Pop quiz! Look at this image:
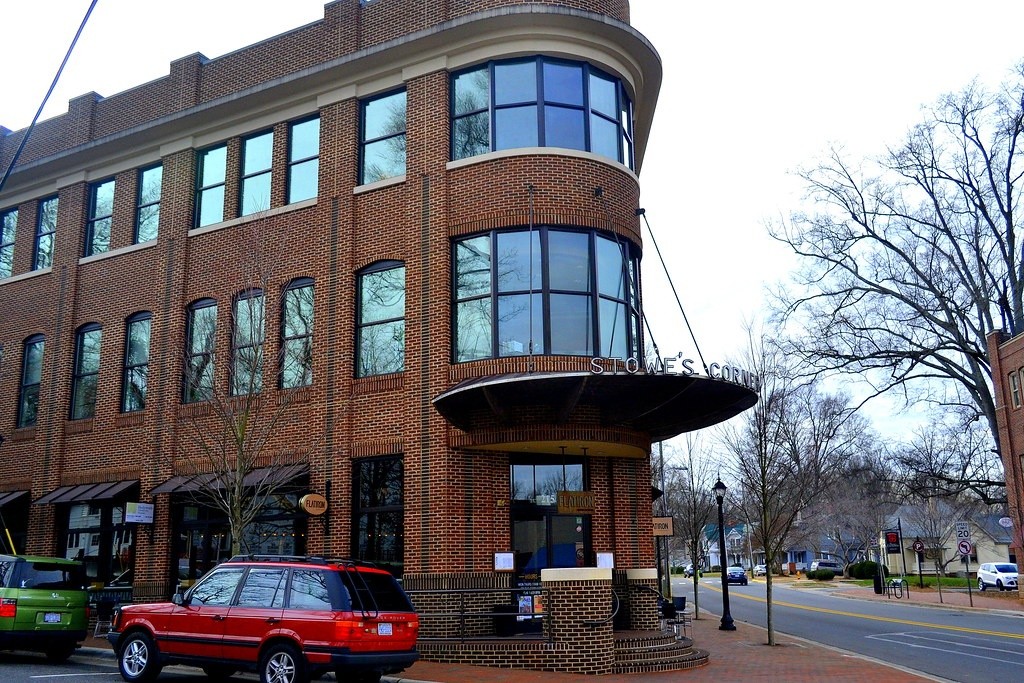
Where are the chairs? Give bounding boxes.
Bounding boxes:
[662,603,687,637]
[672,597,686,637]
[93,602,116,639]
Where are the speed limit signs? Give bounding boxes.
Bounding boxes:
[955,521,970,538]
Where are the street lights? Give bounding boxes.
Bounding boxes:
[663,466,689,599]
[712,469,736,631]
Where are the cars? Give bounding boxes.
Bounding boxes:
[726,568,748,585]
[684,564,702,578]
[751,565,767,575]
[178,559,203,578]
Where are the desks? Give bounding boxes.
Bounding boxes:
[658,611,694,638]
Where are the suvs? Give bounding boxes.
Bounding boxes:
[977,562,1019,591]
[94,554,421,683]
[0,554,90,661]
[810,558,842,574]
